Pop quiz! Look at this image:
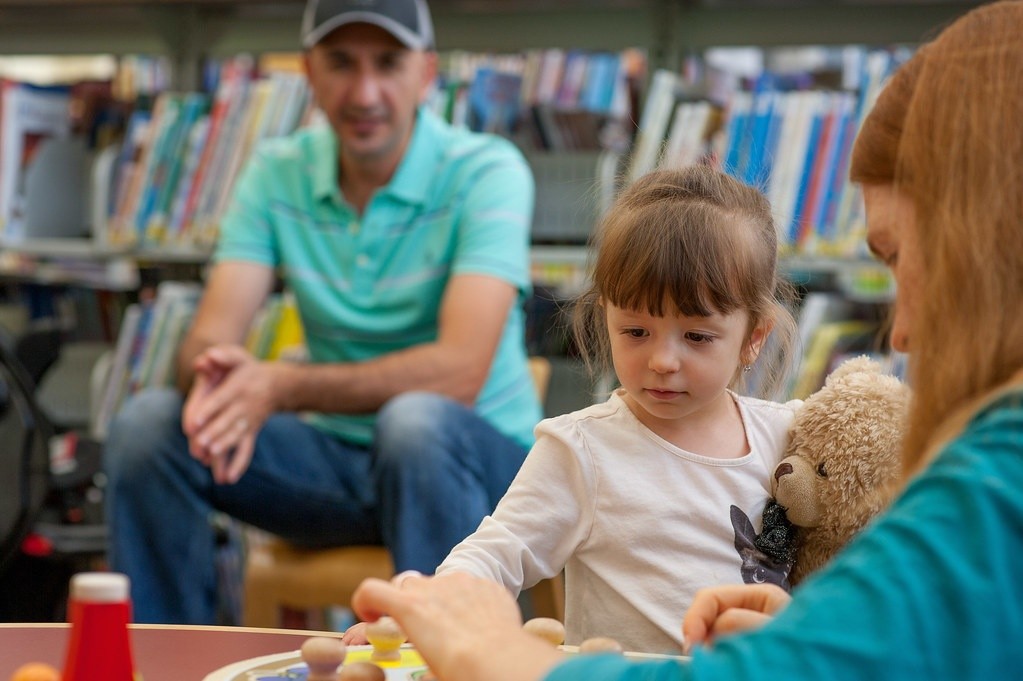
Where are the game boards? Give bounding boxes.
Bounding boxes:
[202,637,694,681]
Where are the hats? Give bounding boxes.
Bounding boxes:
[300,1,438,56]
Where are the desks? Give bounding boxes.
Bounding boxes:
[0,622,693,681]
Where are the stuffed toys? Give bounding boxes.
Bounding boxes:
[758,354,911,583]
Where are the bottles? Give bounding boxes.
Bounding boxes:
[60,572,135,681]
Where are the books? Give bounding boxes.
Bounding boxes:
[427,41,906,403]
[0,55,317,437]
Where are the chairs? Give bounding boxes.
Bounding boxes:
[241,357,564,629]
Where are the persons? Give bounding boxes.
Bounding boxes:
[104,0,544,624]
[353,0,1023,681]
[342,166,807,656]
